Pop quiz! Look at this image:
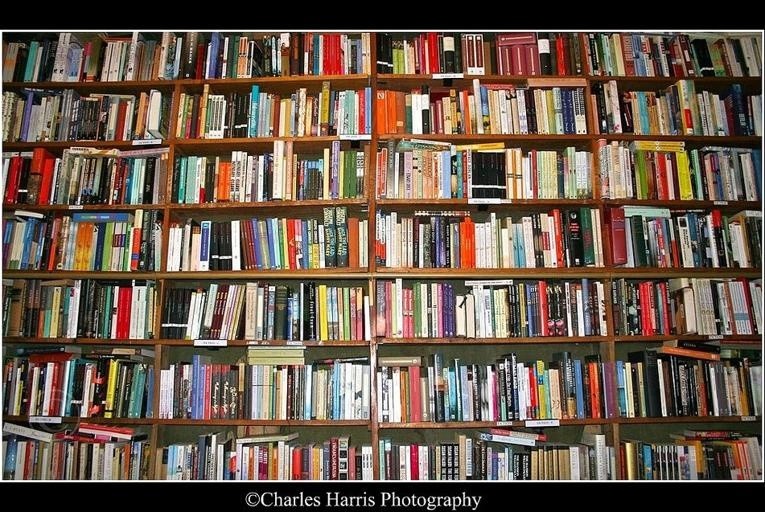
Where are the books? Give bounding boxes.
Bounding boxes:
[584,34,762,76]
[2,279,157,341]
[2,209,162,272]
[183,32,371,79]
[160,281,371,343]
[375,207,604,268]
[154,427,373,479]
[616,341,761,418]
[378,77,588,134]
[590,78,762,138]
[600,139,761,200]
[374,279,607,337]
[166,207,368,271]
[376,138,593,201]
[173,141,370,205]
[2,33,183,82]
[2,147,169,205]
[604,205,762,268]
[2,88,170,140]
[375,352,609,422]
[612,276,762,338]
[175,80,371,138]
[2,421,150,480]
[379,428,616,482]
[2,344,153,418]
[620,429,763,480]
[377,33,586,73]
[159,345,371,421]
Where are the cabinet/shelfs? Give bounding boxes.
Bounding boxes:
[2,28,761,480]
